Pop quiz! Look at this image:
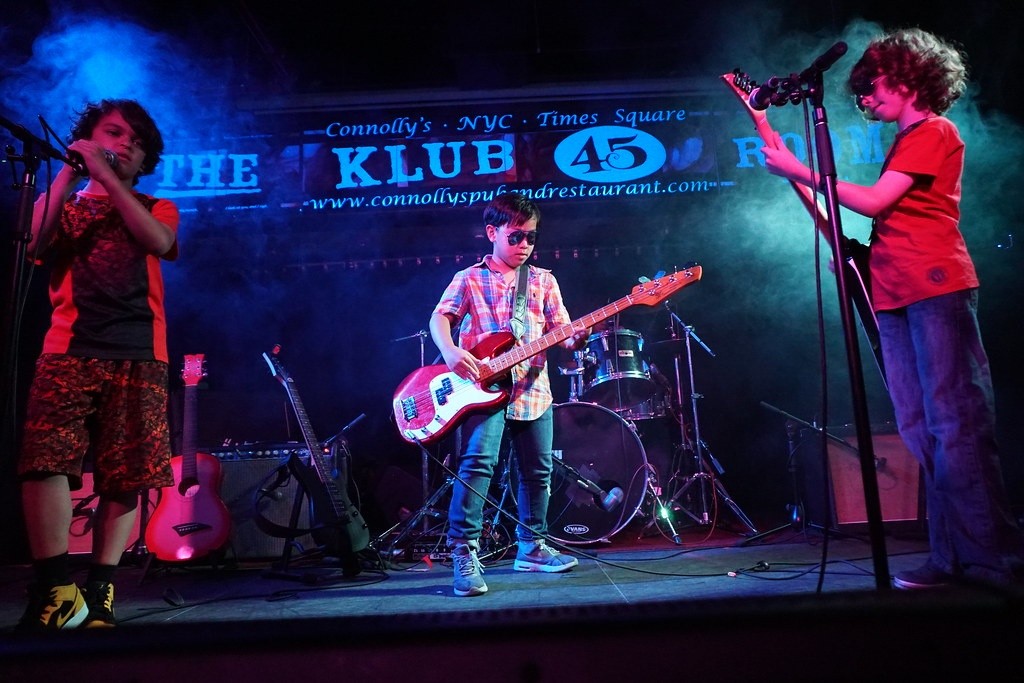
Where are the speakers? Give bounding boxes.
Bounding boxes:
[204,442,337,563]
[63,472,142,557]
[799,421,929,535]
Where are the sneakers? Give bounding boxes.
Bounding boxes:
[16,582,89,631]
[78,580,116,630]
[451,544,488,596]
[514,539,578,572]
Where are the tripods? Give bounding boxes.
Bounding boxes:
[636,296,874,546]
[381,343,600,564]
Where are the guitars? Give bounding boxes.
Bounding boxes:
[391,261,703,445]
[145,352,232,562]
[262,348,371,556]
[720,67,888,393]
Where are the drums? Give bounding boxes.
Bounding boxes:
[576,328,657,411]
[506,402,650,544]
[620,376,684,422]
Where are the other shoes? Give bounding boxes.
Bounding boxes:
[893,557,947,588]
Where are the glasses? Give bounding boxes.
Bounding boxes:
[496,227,537,246]
[857,74,887,96]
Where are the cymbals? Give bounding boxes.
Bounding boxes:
[645,338,703,360]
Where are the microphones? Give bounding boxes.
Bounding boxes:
[71,507,97,517]
[649,363,671,388]
[596,488,626,513]
[65,147,119,169]
[749,77,779,111]
[607,298,616,330]
[258,488,282,501]
[875,459,889,472]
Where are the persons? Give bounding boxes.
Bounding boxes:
[15,98,178,639]
[760,28,1024,598]
[428,193,592,597]
